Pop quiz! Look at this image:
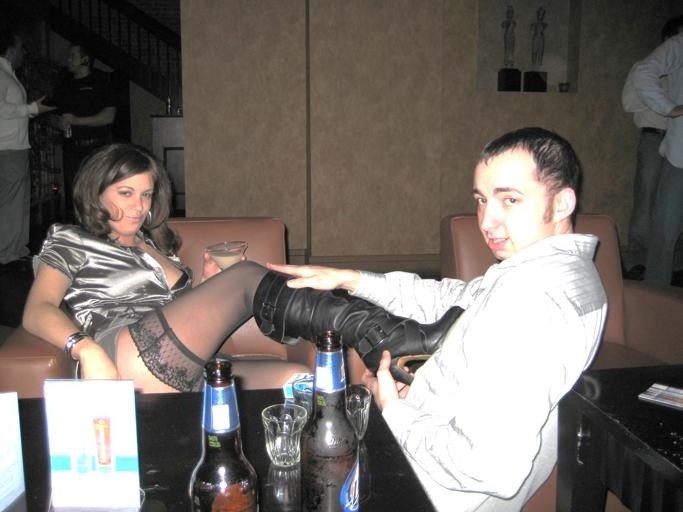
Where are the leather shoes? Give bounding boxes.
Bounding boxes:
[622,264,646,280]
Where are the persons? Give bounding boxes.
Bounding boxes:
[528,7,549,66]
[622,17,683,280]
[266,127,609,512]
[500,4,517,67]
[0,33,57,271]
[22,140,463,394]
[633,33,683,286]
[50,33,117,202]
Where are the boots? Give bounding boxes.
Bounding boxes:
[253,270,464,385]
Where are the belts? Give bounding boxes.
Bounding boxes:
[643,127,666,136]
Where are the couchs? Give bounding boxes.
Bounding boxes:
[439,209,683,511]
[0,215,372,402]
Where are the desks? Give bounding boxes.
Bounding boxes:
[0,379,441,511]
[553,362,683,511]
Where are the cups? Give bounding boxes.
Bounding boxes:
[346,382,371,440]
[265,462,300,511]
[359,441,371,504]
[206,240,248,270]
[291,377,313,418]
[559,83,570,92]
[261,404,306,466]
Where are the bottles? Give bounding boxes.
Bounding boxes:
[297,330,360,511]
[187,360,260,511]
[176,104,182,116]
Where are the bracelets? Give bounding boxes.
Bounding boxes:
[67,333,88,359]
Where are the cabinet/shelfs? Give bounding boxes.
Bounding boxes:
[148,114,185,217]
